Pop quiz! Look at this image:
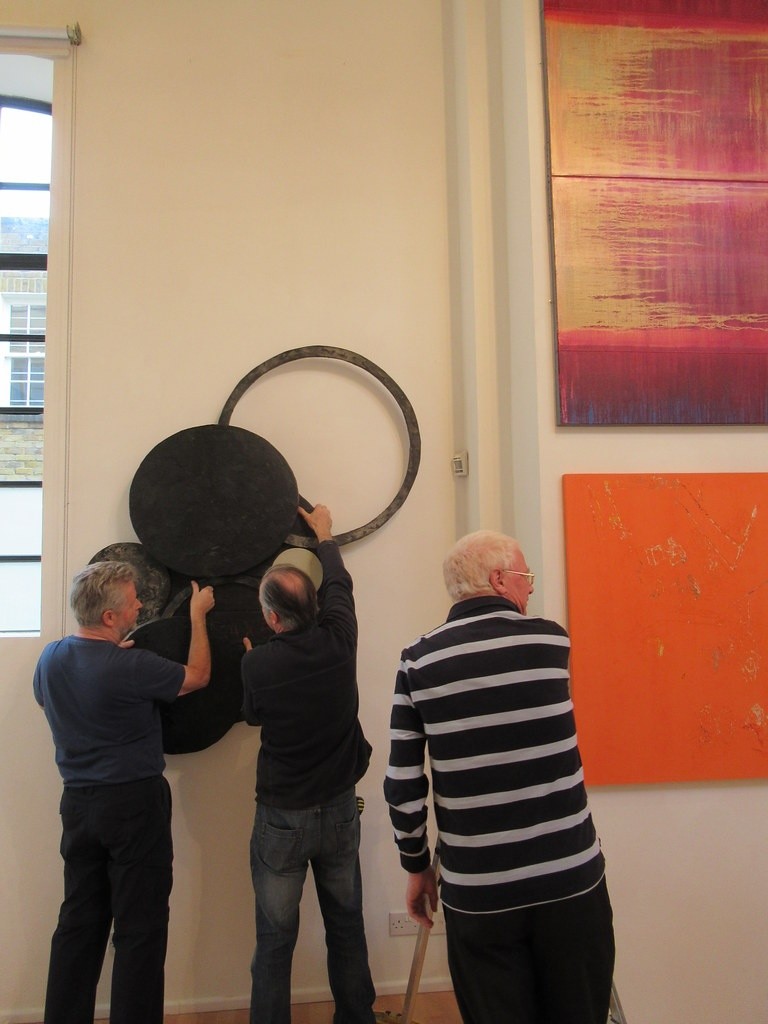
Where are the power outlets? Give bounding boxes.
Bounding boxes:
[388,911,419,936]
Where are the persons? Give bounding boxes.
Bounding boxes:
[383,531,616,1024]
[239,503,377,1024]
[34,561,216,1024]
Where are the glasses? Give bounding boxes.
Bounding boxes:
[504,569,535,585]
